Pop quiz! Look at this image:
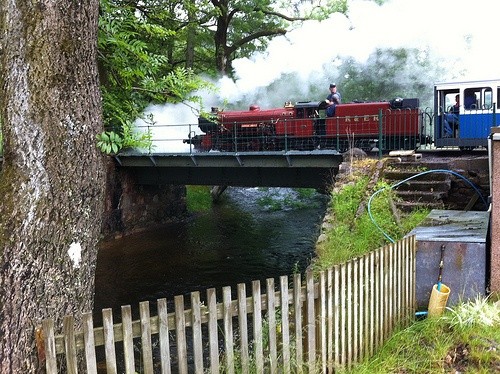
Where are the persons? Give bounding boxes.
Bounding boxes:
[325,82,341,118]
[450,95,460,114]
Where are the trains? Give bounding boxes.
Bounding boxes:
[181,79,500,154]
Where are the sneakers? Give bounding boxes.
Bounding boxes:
[443,134,447,138]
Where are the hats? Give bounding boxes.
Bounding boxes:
[329,82,336,88]
